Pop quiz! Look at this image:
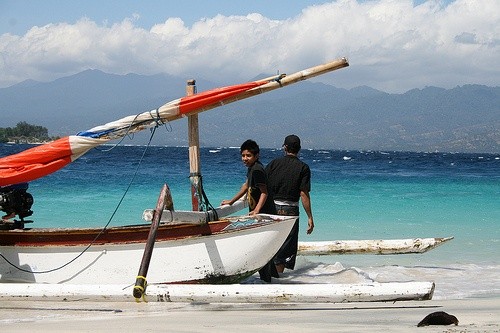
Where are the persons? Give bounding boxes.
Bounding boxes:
[220,140,279,284]
[264,135,314,273]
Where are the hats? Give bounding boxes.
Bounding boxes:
[281,135,301,148]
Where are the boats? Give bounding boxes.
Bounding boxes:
[0,56,350,286]
